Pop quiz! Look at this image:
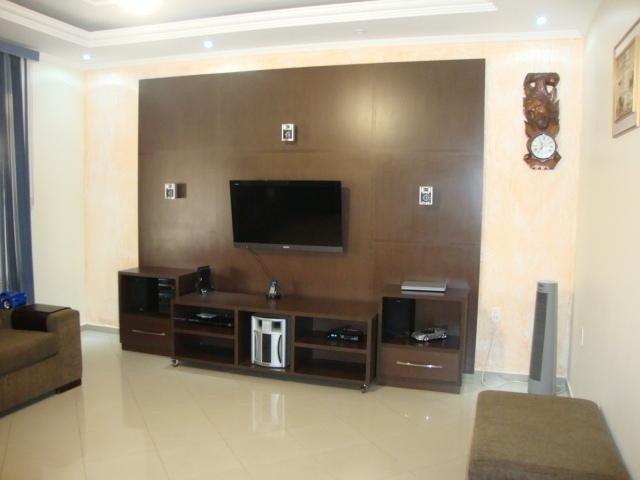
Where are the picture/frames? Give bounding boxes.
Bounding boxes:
[607,14,640,140]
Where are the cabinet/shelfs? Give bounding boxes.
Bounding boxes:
[373,278,470,395]
[169,289,380,394]
[116,265,201,356]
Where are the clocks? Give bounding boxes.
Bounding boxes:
[522,69,561,173]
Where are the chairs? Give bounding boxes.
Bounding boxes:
[1,301,83,420]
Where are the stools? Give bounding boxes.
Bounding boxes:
[467,387,632,479]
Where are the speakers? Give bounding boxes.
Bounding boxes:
[250,314,290,368]
[527,279,558,395]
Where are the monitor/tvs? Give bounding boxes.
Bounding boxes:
[229,180,344,253]
